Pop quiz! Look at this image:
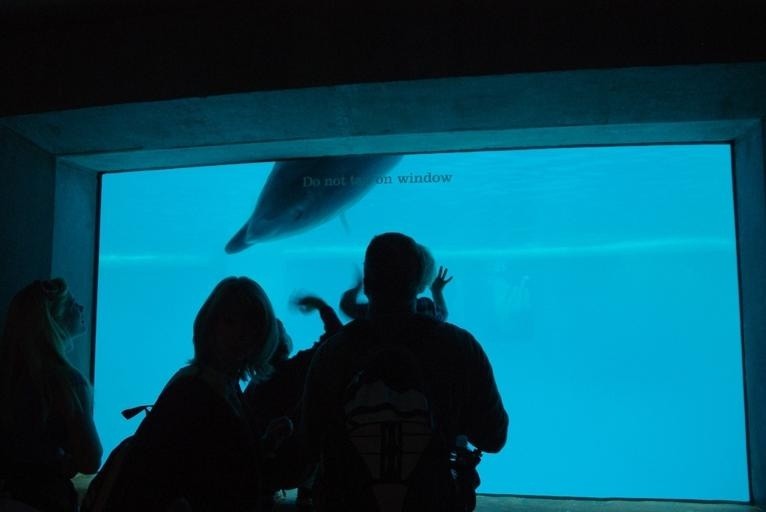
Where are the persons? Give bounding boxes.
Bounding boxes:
[301,233,508,512]
[243,297,343,511]
[1,277,103,511]
[340,268,451,322]
[107,278,280,511]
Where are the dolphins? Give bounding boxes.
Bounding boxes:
[225,151,406,253]
